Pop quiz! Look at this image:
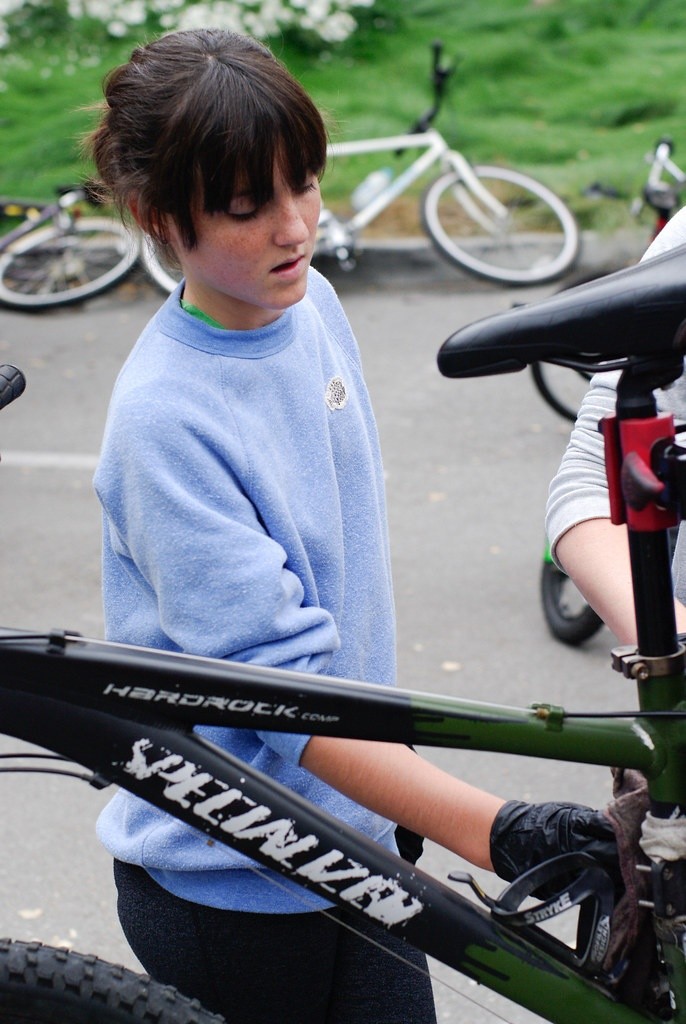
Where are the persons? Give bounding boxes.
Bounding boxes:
[545,207,686,647]
[90,29,620,1024]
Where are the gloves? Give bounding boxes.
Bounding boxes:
[489,797,624,904]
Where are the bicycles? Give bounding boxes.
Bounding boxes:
[0,182,143,310]
[530,137,685,425]
[1,242,685,1022]
[140,42,583,299]
